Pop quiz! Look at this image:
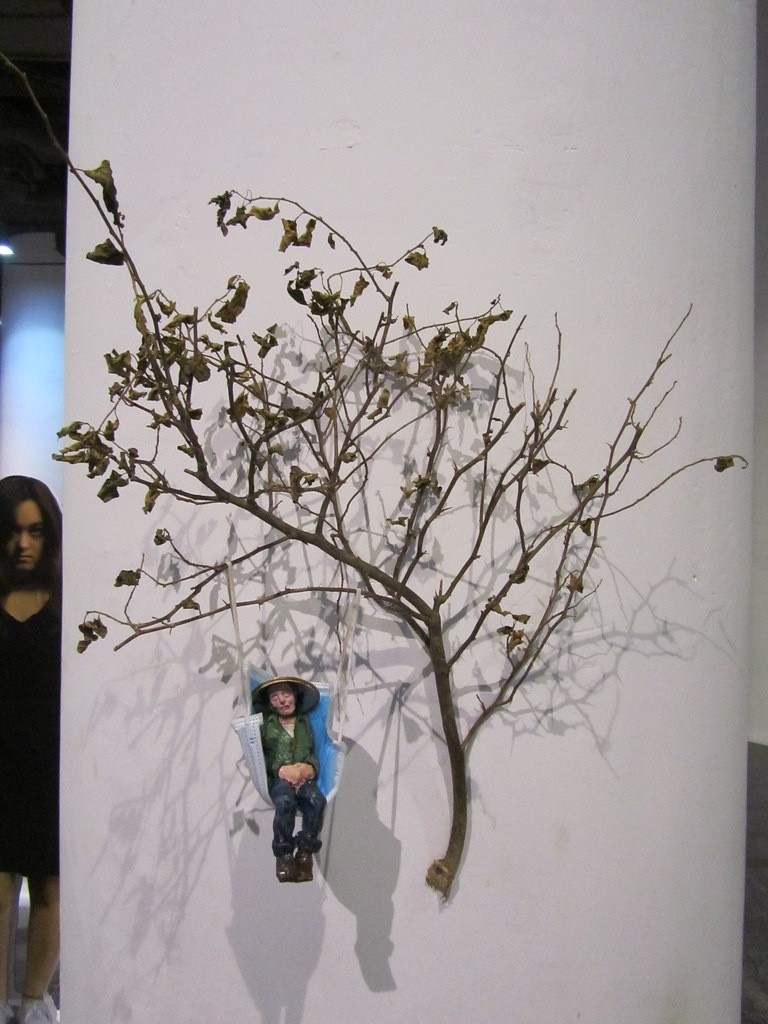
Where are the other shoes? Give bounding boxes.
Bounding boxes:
[21,992,57,1024]
[0,1000,16,1024]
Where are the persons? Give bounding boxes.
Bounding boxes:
[0,476,62,1024]
[253,676,327,882]
